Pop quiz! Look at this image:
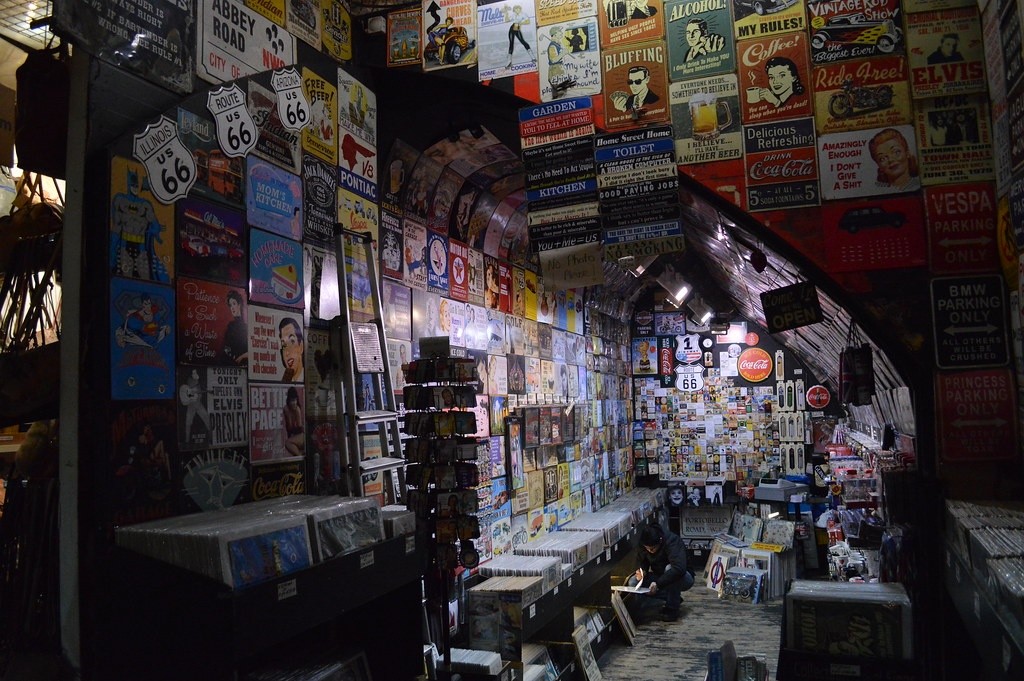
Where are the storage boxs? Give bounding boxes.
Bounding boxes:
[110,526,425,664]
[754,483,810,501]
[776,578,926,681]
[940,527,1024,681]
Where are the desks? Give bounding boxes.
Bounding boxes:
[747,495,831,572]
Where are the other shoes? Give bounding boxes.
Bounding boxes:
[662,613,677,622]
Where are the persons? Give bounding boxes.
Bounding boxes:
[637,523,696,621]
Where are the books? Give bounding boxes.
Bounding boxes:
[945,498,1024,630]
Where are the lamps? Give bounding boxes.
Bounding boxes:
[625,255,730,335]
